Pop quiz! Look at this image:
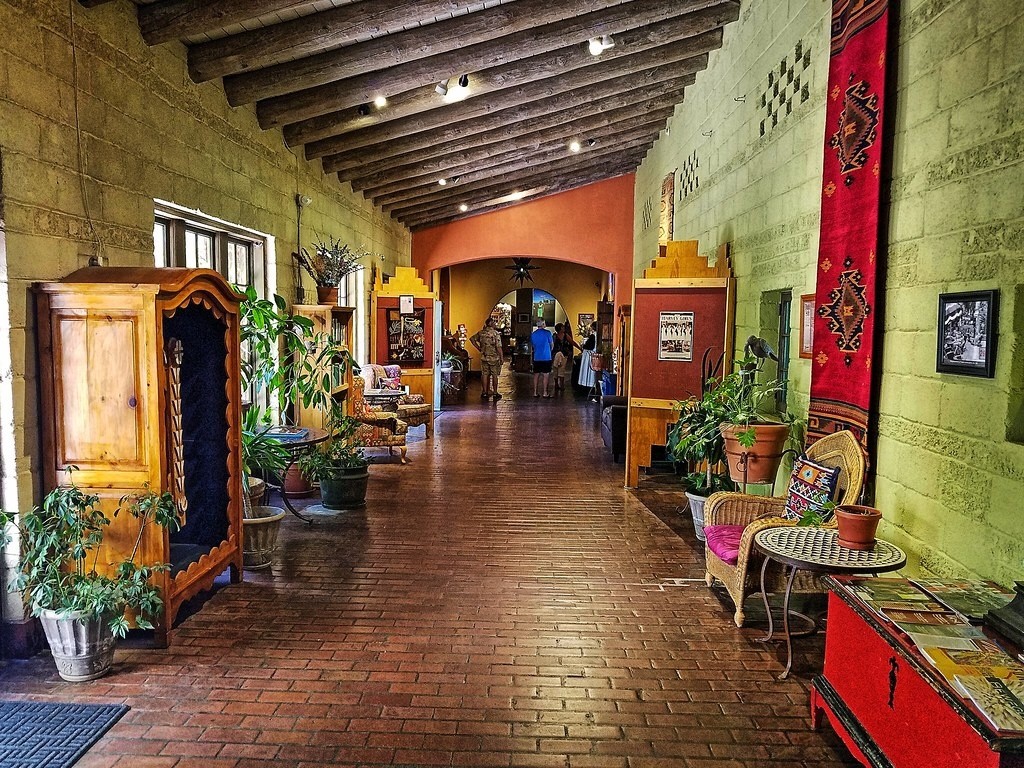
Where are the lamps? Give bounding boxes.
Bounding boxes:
[434,78,450,96]
[587,139,596,146]
[452,176,460,184]
[358,103,371,116]
[458,74,469,87]
[588,34,616,56]
[502,257,542,289]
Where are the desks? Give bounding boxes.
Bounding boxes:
[809,573,1024,768]
[255,425,330,526]
[362,388,407,414]
[753,525,907,680]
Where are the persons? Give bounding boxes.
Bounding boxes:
[530,317,554,397]
[553,323,582,393]
[470,318,503,398]
[662,323,692,337]
[577,322,597,388]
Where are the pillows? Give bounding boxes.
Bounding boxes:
[378,376,405,406]
[780,455,841,525]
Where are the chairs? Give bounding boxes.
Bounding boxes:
[702,430,866,629]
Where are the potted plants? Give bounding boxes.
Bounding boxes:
[0,464,182,684]
[230,233,370,572]
[665,345,727,541]
[591,346,612,369]
[799,492,883,551]
[666,334,812,492]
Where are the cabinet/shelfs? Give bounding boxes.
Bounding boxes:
[290,304,358,455]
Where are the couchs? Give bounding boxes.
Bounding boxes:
[353,363,432,464]
[600,395,629,465]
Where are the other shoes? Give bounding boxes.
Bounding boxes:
[493,393,502,399]
[550,391,558,397]
[481,393,489,400]
[533,392,539,397]
[543,393,549,397]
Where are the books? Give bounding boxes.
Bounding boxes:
[266,426,309,437]
[834,575,1024,734]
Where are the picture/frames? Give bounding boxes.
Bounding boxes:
[399,294,414,314]
[578,313,595,327]
[935,288,1003,379]
[657,310,694,362]
[518,312,531,324]
[798,293,817,359]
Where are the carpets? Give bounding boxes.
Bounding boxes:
[0,700,132,768]
